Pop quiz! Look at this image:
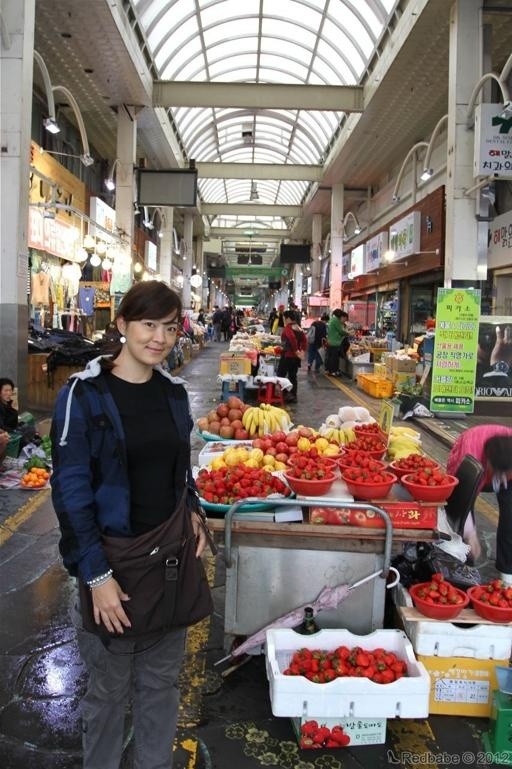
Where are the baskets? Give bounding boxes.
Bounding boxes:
[283,427,459,502]
[410,583,512,623]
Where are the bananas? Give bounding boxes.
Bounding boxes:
[241,403,292,438]
[327,429,356,446]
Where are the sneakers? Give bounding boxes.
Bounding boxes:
[328,369,341,376]
[501,570,512,583]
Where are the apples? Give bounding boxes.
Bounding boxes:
[198,396,248,440]
[253,427,313,461]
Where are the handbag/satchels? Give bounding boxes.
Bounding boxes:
[78,485,213,636]
[340,337,350,359]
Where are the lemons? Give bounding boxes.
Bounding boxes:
[211,446,286,472]
[22,467,50,487]
[296,436,342,455]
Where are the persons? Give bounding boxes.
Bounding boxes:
[477,323,512,388]
[50,280,214,769]
[446,424,512,588]
[0,378,18,432]
[198,306,348,404]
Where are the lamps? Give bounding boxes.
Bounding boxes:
[141,206,185,256]
[391,113,448,203]
[105,159,122,190]
[465,53,512,120]
[344,212,361,237]
[33,45,93,166]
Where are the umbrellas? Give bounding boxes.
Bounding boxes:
[213,566,400,668]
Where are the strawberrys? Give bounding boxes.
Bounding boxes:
[417,573,464,606]
[471,579,512,608]
[395,453,437,470]
[300,720,350,750]
[340,450,380,467]
[407,467,450,486]
[344,464,392,483]
[195,466,290,504]
[288,447,334,479]
[284,646,408,684]
[344,436,385,452]
[355,422,382,434]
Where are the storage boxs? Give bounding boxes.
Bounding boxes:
[290,717,387,750]
[264,628,429,720]
[393,580,512,661]
[357,353,416,398]
[219,357,251,374]
[310,502,437,530]
[419,657,509,718]
[39,418,53,439]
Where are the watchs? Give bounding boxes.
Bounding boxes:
[488,361,512,373]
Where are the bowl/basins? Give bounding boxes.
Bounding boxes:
[466,585,512,623]
[196,492,294,513]
[409,582,470,620]
[194,421,295,443]
[282,428,459,502]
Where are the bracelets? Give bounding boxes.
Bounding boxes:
[86,569,114,587]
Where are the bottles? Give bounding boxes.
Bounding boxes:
[302,606,319,635]
